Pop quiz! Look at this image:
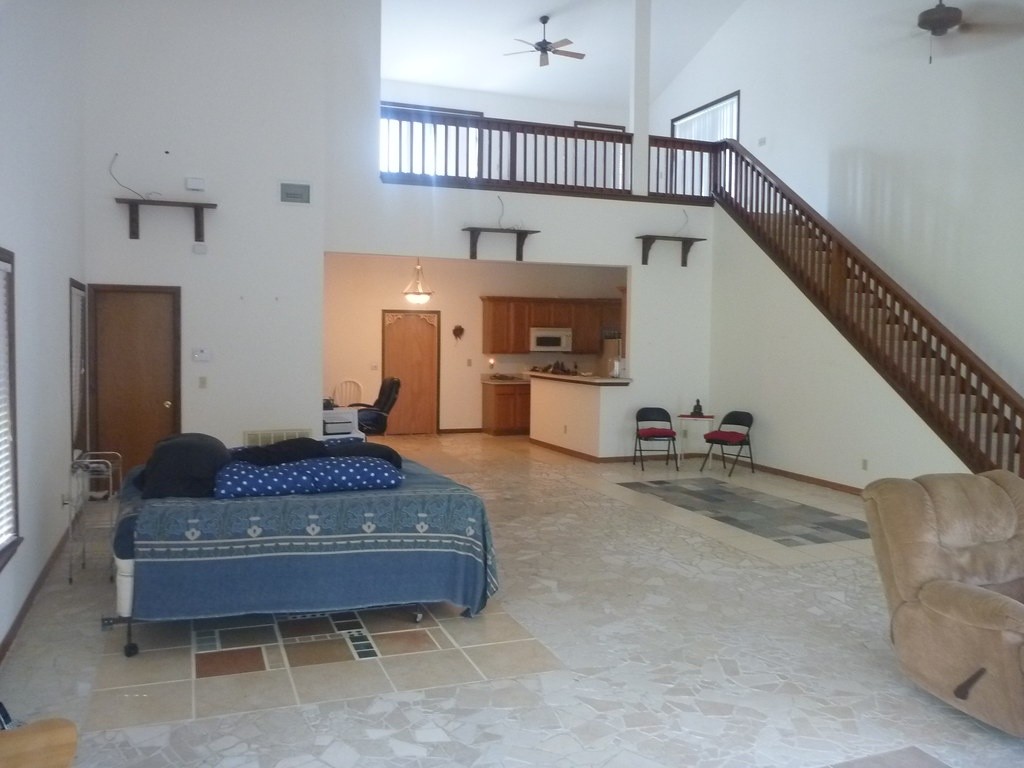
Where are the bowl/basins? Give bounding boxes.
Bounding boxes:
[603,328,621,338]
[522,372,531,381]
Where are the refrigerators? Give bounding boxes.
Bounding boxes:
[603,339,622,377]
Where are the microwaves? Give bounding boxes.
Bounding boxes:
[530,327,573,352]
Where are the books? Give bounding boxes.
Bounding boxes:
[325,416,352,434]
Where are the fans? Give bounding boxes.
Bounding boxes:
[505,16,585,67]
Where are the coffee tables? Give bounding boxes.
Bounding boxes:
[677,415,713,470]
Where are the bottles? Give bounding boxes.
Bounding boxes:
[574,359,578,374]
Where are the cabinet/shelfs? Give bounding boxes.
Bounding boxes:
[484,296,621,353]
[482,384,530,435]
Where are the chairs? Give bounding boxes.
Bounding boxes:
[632,407,681,470]
[335,377,400,435]
[860,471,1024,738]
[701,412,758,477]
[0,699,76,768]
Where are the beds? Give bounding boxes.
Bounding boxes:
[117,454,489,615]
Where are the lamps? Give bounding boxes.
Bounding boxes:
[402,256,434,305]
[916,0,961,36]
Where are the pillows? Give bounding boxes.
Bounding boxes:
[144,434,402,495]
